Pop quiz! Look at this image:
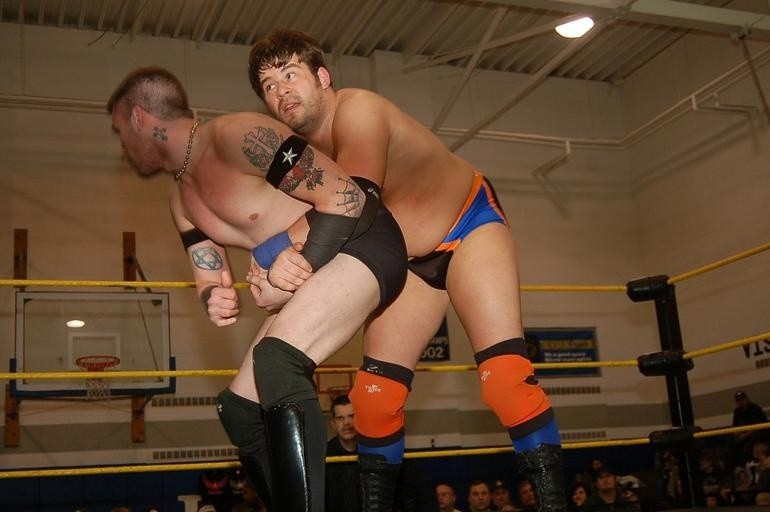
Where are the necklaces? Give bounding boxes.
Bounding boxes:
[173,121,200,182]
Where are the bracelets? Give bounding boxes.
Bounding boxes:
[251,229,295,273]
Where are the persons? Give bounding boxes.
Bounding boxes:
[730,389,769,448]
[247,30,578,512]
[324,393,364,512]
[437,479,536,510]
[201,470,266,510]
[105,65,408,511]
[573,444,768,511]
